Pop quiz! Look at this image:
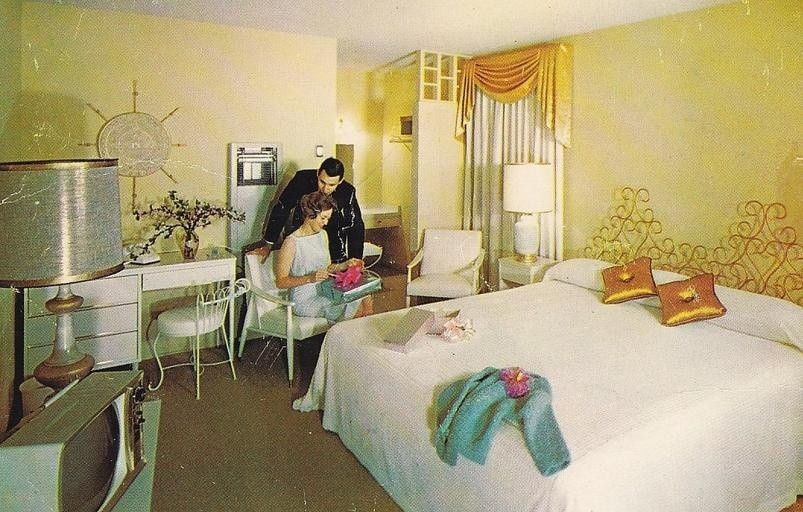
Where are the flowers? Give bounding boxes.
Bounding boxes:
[131,188,249,255]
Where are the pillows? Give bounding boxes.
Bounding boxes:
[600,255,726,327]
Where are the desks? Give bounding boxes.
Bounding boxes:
[0,397,164,512]
[2,244,237,386]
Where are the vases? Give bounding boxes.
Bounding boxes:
[177,229,198,260]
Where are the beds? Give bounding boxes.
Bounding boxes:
[326,254,803,512]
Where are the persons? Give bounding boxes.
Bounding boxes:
[276,190,373,325]
[244,157,365,264]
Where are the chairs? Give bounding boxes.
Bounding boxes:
[397,225,488,308]
[145,236,333,402]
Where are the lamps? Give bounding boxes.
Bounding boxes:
[498,160,555,263]
[1,156,126,413]
